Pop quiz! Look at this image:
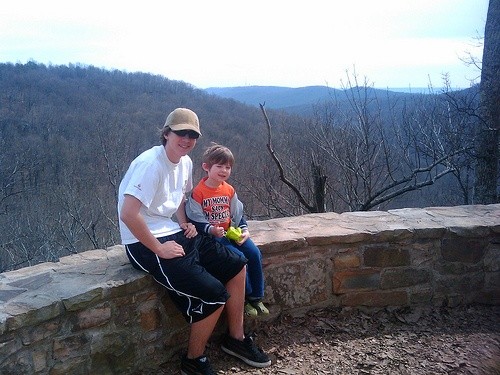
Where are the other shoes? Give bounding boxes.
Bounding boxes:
[244,303,257,317]
[181,350,218,375]
[221,330,271,367]
[256,301,270,317]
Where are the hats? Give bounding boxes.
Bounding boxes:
[163,108,203,137]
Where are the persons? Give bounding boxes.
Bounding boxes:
[118,108,271,375]
[186,145,269,317]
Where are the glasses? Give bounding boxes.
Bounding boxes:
[174,130,199,138]
